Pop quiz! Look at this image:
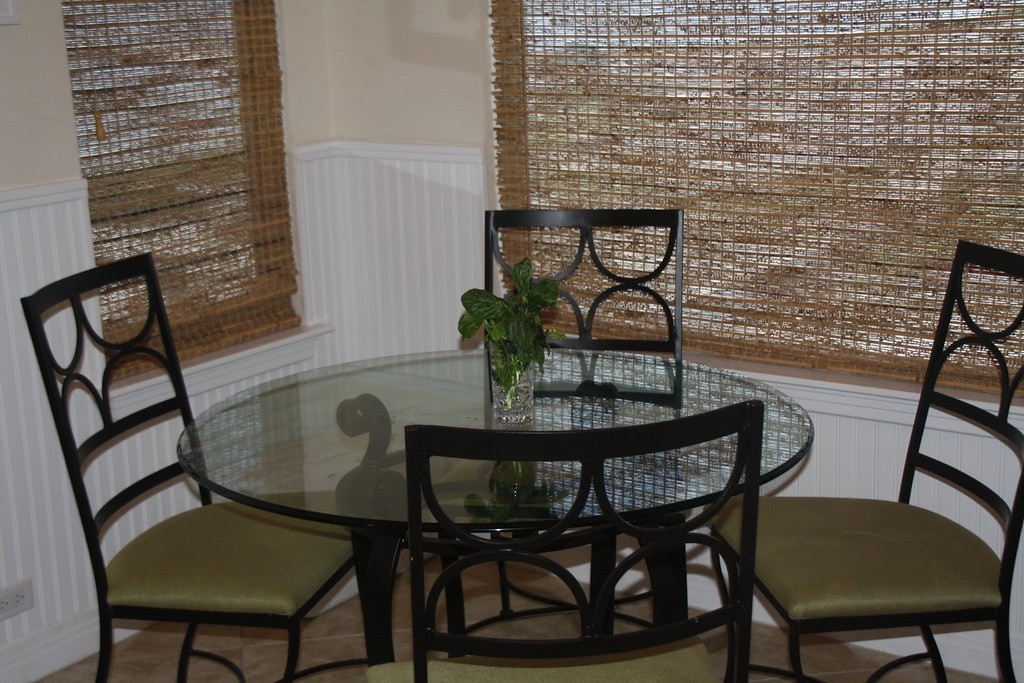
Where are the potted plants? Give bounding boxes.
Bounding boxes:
[455,259,564,421]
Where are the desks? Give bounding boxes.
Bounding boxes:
[181,344,813,683]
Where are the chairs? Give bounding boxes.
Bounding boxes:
[26,250,355,683]
[702,241,1023,683]
[358,396,763,683]
[466,204,684,357]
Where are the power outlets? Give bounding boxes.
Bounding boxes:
[1,578,35,623]
